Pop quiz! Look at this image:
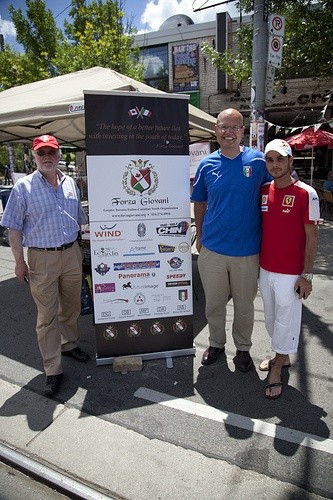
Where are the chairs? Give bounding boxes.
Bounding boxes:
[315,187,333,221]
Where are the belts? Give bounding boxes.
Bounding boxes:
[30,236,79,252]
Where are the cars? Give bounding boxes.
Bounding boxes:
[78,200,94,314]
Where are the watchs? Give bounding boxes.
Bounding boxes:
[300,272,313,281]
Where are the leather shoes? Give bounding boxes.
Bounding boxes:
[201,346,225,365]
[235,350,252,371]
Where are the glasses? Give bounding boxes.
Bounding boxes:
[216,123,244,132]
[36,150,56,156]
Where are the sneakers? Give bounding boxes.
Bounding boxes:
[43,373,64,395]
[61,347,89,362]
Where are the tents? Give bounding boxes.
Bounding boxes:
[1,64,218,154]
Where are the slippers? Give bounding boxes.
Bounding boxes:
[264,377,284,399]
[259,358,291,371]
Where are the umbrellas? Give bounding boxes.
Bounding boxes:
[283,127,332,150]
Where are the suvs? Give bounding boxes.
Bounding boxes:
[0,185,26,248]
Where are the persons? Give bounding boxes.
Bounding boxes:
[259,139,320,398]
[190,108,275,371]
[0,134,89,397]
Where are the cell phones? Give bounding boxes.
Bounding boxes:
[296,286,305,298]
[24,276,29,284]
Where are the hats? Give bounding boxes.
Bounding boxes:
[263,139,293,161]
[32,134,59,151]
